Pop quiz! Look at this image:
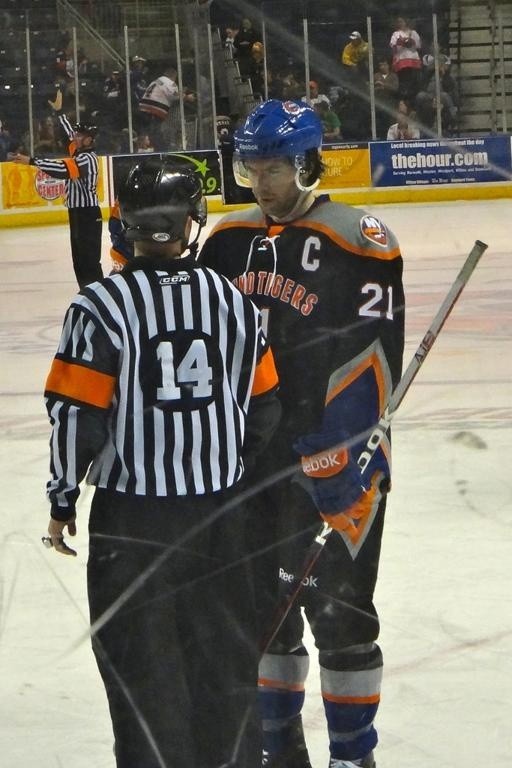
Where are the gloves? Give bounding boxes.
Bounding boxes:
[285,428,378,531]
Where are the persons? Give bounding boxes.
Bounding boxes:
[42,158,284,767]
[15,88,102,286]
[1,7,460,163]
[202,98,401,767]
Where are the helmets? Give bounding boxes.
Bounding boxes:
[232,97,324,164]
[72,121,98,135]
[117,152,210,244]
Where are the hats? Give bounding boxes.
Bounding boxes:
[348,31,361,41]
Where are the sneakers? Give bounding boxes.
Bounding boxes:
[259,747,313,768]
[328,749,378,768]
